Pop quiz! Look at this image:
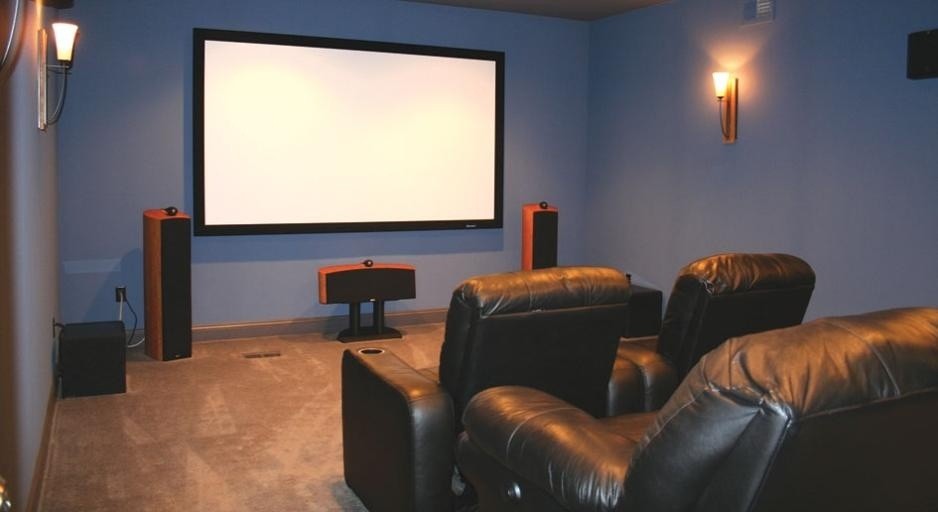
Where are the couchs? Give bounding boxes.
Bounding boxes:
[340,267,631,512]
[615,253,817,410]
[452,306,938,511]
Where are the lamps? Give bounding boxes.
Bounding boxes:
[713,69,738,144]
[37,20,79,130]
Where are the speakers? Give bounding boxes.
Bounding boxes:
[144,207,193,362]
[317,263,417,304]
[905,29,937,80]
[520,202,558,271]
[58,320,127,399]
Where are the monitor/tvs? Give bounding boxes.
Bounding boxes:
[191,23,507,238]
